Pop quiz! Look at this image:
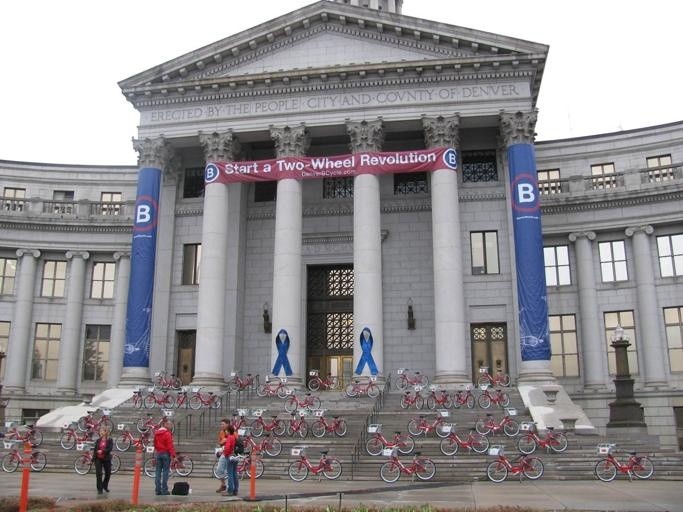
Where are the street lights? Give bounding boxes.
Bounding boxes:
[603,323,647,428]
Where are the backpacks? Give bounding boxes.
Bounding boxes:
[232,434,244,455]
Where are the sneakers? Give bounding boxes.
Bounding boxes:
[98,489,109,494]
[216,485,238,496]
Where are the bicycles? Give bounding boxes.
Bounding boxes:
[287,411,309,439]
[395,367,430,392]
[476,412,520,437]
[476,367,511,389]
[365,423,415,456]
[2,423,44,449]
[344,376,381,398]
[453,389,476,409]
[595,442,655,482]
[188,385,222,411]
[399,388,425,410]
[60,408,115,451]
[115,427,155,453]
[426,388,453,410]
[210,407,287,480]
[74,443,122,476]
[132,370,189,410]
[135,409,175,434]
[407,413,452,438]
[287,444,343,482]
[143,449,193,479]
[308,368,340,392]
[486,453,545,483]
[380,445,437,483]
[311,409,348,438]
[439,426,491,457]
[284,390,322,414]
[227,367,291,400]
[477,389,511,409]
[516,421,568,455]
[2,447,47,473]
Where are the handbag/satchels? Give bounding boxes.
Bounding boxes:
[172,482,188,495]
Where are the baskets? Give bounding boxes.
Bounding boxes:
[230,373,237,377]
[164,411,173,416]
[384,449,397,457]
[148,386,154,392]
[599,447,609,454]
[77,444,86,451]
[4,422,14,449]
[309,372,318,376]
[367,427,378,433]
[465,384,487,390]
[480,368,488,374]
[299,410,324,416]
[117,424,125,430]
[397,370,403,374]
[509,410,517,415]
[181,386,200,392]
[371,378,376,381]
[155,373,161,377]
[281,378,293,395]
[292,449,303,455]
[415,386,436,391]
[519,424,530,430]
[235,409,263,435]
[440,412,451,432]
[489,449,499,455]
[147,447,155,452]
[103,411,112,415]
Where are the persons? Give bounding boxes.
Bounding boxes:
[215,419,238,492]
[88,426,112,495]
[221,424,240,496]
[153,417,178,495]
